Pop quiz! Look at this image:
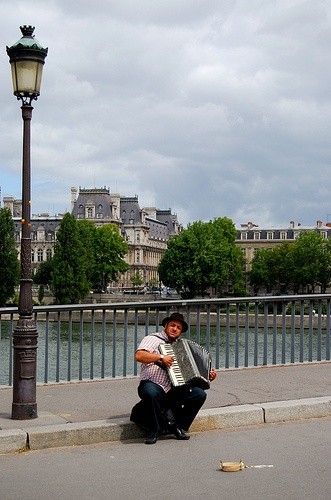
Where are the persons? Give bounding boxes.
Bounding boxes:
[134,312,217,444]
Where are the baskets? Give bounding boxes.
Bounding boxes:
[220,458,244,471]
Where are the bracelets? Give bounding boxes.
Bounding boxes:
[157,355,164,365]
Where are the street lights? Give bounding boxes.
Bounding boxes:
[5,25,50,420]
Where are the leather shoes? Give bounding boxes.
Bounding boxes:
[143,430,160,444]
[168,425,191,440]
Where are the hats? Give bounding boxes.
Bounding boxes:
[162,313,188,333]
